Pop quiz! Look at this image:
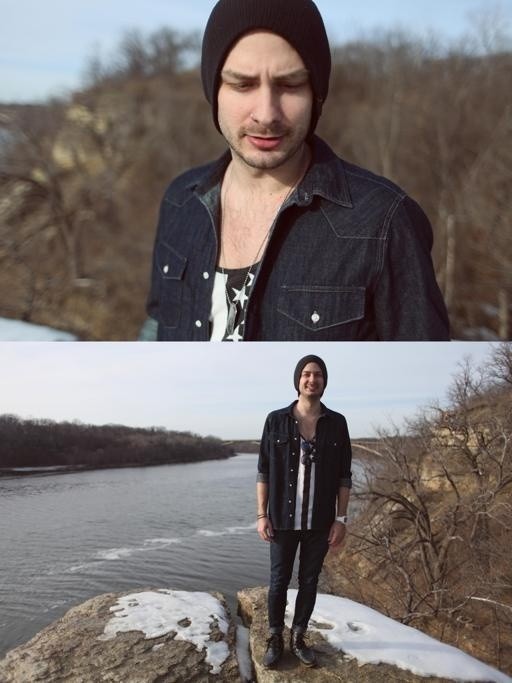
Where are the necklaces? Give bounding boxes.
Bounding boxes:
[298,414,316,440]
[218,150,309,337]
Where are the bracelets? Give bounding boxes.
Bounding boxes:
[256,515,267,519]
[255,513,267,517]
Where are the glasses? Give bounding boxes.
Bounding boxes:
[301,441,311,466]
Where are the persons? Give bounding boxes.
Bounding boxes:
[254,353,353,671]
[142,0,454,341]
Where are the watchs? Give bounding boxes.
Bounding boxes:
[334,515,348,524]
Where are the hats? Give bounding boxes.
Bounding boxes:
[294,355,327,396]
[202,0,330,138]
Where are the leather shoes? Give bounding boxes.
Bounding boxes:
[290,631,317,668]
[263,633,283,669]
